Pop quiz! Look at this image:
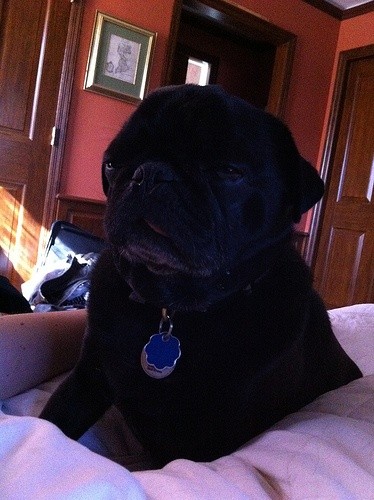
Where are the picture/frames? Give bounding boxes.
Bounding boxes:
[82,6,159,107]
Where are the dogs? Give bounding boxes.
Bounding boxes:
[39,82,366,473]
